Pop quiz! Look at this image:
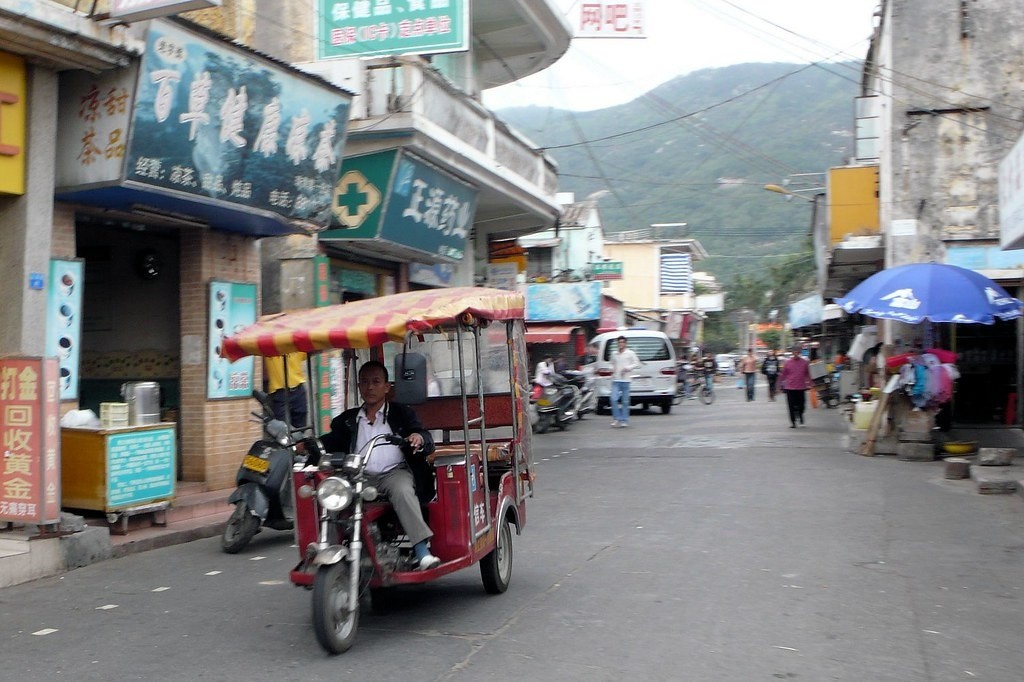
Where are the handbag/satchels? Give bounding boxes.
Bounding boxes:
[810,386,818,408]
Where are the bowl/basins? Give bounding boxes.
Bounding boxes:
[942,442,978,453]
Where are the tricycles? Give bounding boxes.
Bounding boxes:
[216,286,539,653]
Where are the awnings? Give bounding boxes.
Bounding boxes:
[524,325,580,343]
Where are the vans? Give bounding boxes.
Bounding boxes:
[580,326,678,414]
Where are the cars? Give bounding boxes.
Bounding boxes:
[715,353,737,377]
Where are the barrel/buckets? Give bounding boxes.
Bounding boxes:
[854,400,879,429]
[121,381,160,426]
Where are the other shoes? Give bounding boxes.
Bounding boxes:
[611,419,621,425]
[621,421,628,426]
[791,423,795,428]
[800,415,804,423]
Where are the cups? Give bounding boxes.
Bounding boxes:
[213,344,222,363]
[214,318,225,334]
[58,274,75,296]
[215,290,227,311]
[57,304,74,326]
[58,336,73,360]
[212,370,223,390]
[60,367,71,390]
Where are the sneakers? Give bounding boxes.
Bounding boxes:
[419,554,441,571]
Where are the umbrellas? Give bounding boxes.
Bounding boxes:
[833,259,1024,347]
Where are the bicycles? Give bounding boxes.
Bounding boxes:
[672,359,715,407]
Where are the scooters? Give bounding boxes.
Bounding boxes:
[221,389,314,554]
[529,369,597,433]
[812,375,841,408]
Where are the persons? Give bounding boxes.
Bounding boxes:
[609,336,643,428]
[676,351,718,392]
[776,345,812,428]
[835,350,847,363]
[762,349,780,402]
[554,352,568,374]
[736,347,761,402]
[535,354,555,379]
[295,360,441,572]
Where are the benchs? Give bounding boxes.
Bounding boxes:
[413,392,514,461]
